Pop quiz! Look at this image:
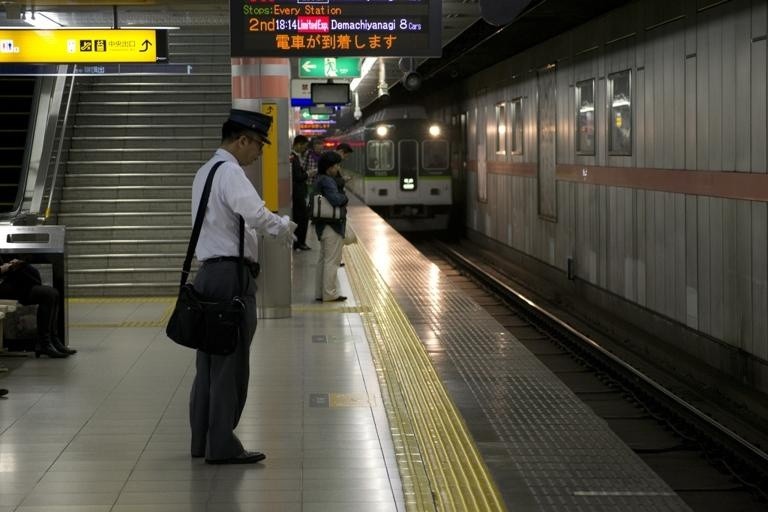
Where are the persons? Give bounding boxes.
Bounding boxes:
[189,109,298,466]
[291,135,353,301]
[0,259,76,358]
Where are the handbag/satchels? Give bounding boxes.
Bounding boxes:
[165,283,246,357]
[309,193,344,221]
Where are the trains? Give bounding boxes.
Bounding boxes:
[312,105,459,233]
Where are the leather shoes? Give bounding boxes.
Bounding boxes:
[316,294,348,303]
[206,450,266,465]
[34,338,77,358]
[293,243,311,250]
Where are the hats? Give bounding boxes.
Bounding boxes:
[227,107,276,146]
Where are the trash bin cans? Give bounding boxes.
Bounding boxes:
[257,225,292,307]
[1,224,68,357]
